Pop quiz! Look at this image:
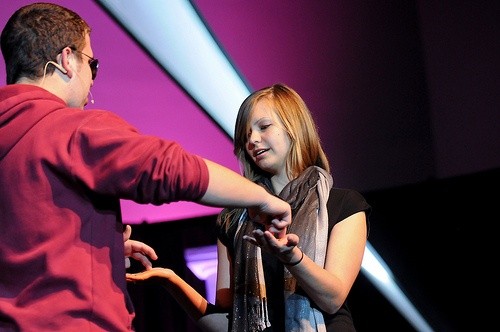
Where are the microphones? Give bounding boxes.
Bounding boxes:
[51,46,94,104]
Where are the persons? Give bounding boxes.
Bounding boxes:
[0,2,292,332]
[125,83,371,332]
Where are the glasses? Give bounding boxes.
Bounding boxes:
[56,45,100,80]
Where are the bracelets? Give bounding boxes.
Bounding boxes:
[283,252,303,266]
